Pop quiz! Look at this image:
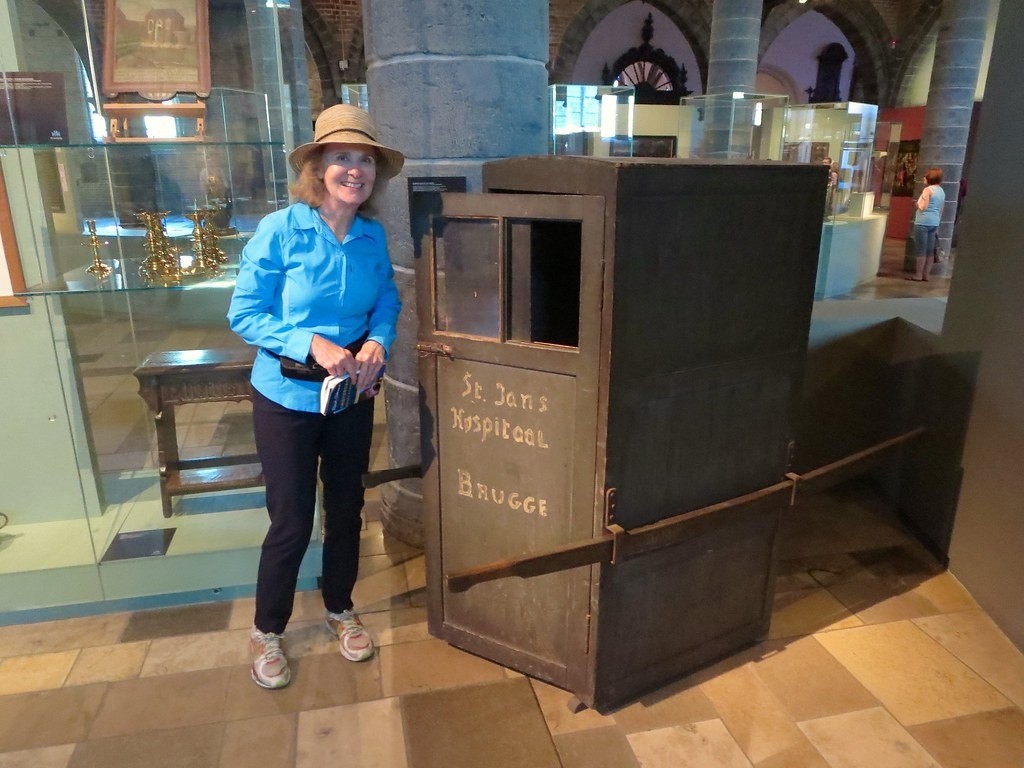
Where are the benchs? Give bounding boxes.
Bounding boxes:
[134,344,264,518]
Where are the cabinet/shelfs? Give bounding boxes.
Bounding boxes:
[0,141,286,300]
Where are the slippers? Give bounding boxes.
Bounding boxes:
[905,273,930,281]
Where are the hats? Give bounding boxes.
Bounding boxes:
[288,104,405,181]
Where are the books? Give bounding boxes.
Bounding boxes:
[320,363,386,416]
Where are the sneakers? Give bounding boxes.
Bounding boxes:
[247,624,291,689]
[325,607,375,662]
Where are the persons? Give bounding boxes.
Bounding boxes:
[226,104,404,690]
[904,168,946,282]
[815,153,840,217]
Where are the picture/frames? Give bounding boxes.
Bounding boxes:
[101,0,213,98]
